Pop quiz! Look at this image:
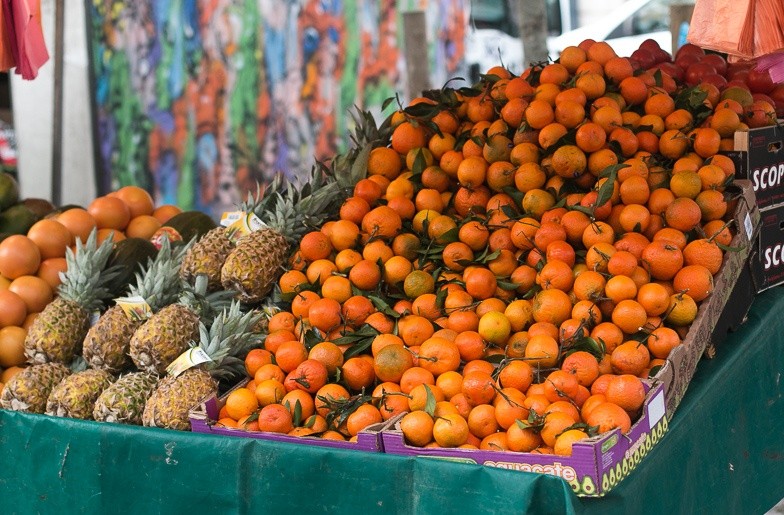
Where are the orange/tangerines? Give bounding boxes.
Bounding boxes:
[0,186,183,396]
[214,38,783,458]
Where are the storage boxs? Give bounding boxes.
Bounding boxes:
[720,115,784,209]
[754,200,784,296]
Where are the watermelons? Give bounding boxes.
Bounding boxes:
[0,172,87,243]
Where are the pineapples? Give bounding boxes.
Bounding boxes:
[0,102,383,430]
